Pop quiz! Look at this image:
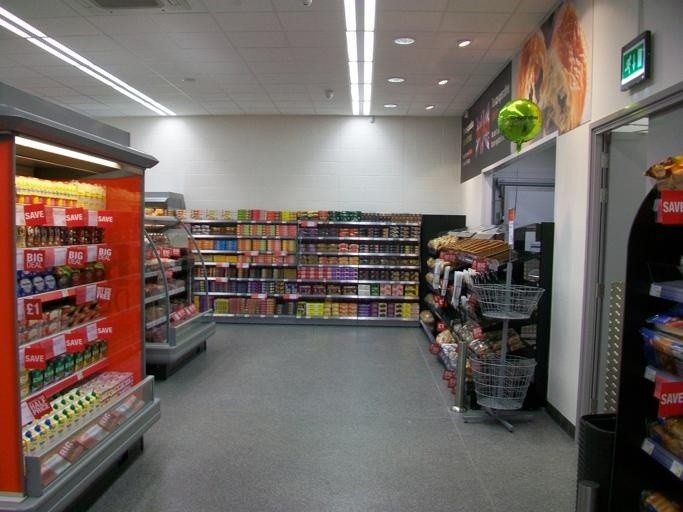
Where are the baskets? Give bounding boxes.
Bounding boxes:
[447,238,513,265]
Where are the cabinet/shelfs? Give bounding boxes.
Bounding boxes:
[0,80,162,510]
[178,220,418,328]
[417,214,553,410]
[141,191,215,383]
[601,167,683,511]
